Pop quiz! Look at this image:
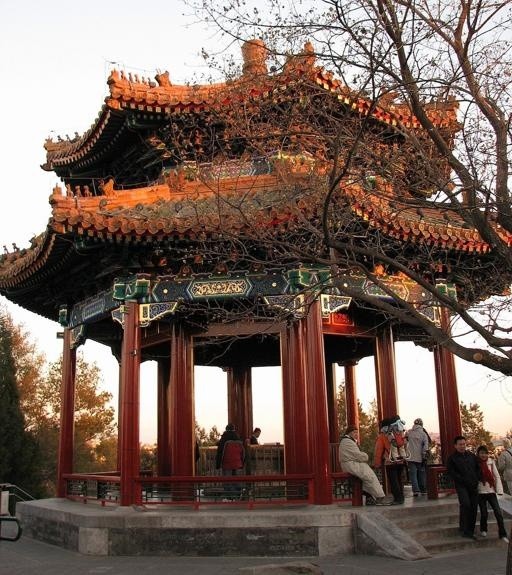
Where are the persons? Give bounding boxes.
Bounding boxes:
[250,427,262,445]
[497,438,512,497]
[373,417,405,505]
[422,427,433,485]
[447,433,481,540]
[475,444,510,544]
[387,416,410,463]
[407,418,428,496]
[339,424,394,506]
[214,422,246,503]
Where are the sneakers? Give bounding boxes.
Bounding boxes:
[367,498,393,506]
[223,498,236,502]
[391,455,409,463]
[501,536,509,544]
[414,493,419,497]
[481,531,487,537]
[239,489,246,500]
[463,533,477,539]
